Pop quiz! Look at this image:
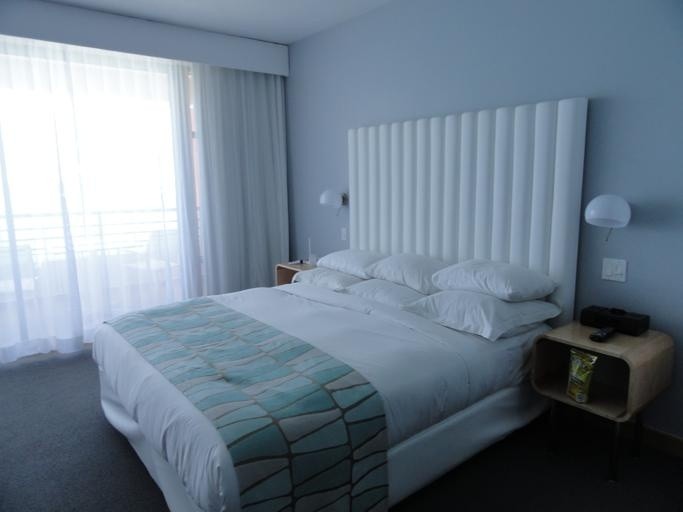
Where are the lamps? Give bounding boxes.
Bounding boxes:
[585,194,632,230]
[318,189,348,209]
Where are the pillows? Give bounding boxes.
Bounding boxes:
[367,254,452,296]
[292,268,364,293]
[342,277,426,311]
[431,257,559,302]
[405,289,564,344]
[316,246,387,279]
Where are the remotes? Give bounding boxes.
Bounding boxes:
[589,326,618,342]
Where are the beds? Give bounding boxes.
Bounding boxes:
[90,97,588,511]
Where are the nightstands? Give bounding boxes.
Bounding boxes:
[275,259,313,286]
[530,319,674,424]
